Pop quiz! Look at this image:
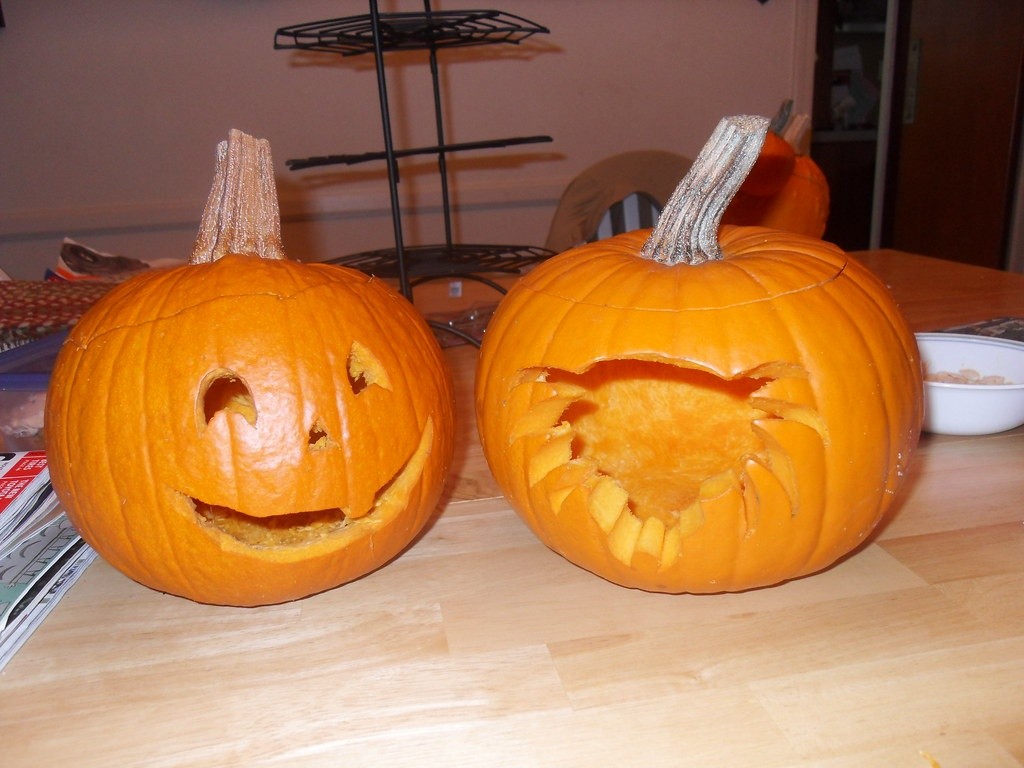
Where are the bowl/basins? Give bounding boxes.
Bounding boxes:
[899,332,1024,436]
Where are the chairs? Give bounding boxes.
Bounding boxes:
[544,149,695,248]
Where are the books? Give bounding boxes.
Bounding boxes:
[0,451,99,676]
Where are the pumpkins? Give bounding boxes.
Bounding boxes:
[719,114,830,238]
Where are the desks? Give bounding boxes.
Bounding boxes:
[0,249,1024,768]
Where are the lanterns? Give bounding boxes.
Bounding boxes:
[473,114,925,595]
[41,130,457,606]
